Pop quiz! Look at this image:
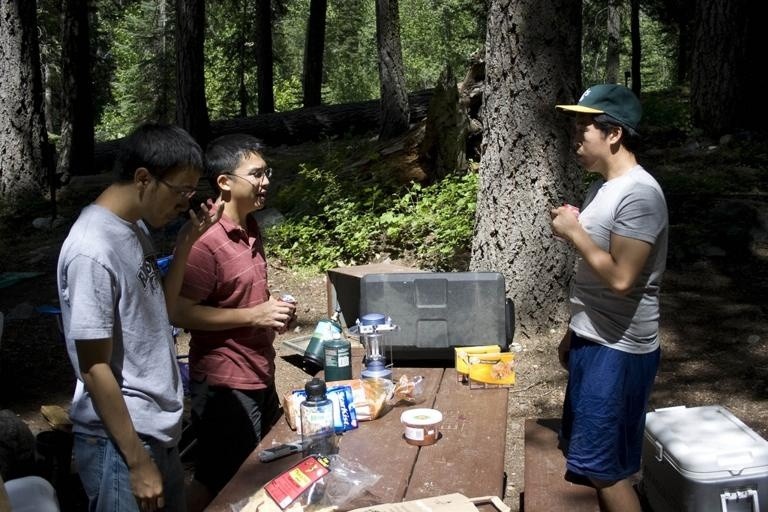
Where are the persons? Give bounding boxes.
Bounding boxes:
[549,83,669,512]
[169,133,298,512]
[56,122,225,512]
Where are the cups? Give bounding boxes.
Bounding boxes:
[400,406,444,446]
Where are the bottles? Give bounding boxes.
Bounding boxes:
[303,311,343,370]
[300,376,336,462]
[324,330,352,383]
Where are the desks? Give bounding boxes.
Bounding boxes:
[206,361,507,512]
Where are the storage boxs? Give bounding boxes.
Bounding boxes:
[316,264,414,350]
[354,269,514,359]
[640,401,767,512]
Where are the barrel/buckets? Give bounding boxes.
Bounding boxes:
[31,429,83,495]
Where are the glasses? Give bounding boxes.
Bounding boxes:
[222,167,273,179]
[153,175,197,201]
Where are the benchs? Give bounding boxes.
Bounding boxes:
[525,408,602,512]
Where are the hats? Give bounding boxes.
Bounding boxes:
[554,83,644,133]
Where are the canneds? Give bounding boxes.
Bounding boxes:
[551,203,580,244]
[270,294,298,332]
